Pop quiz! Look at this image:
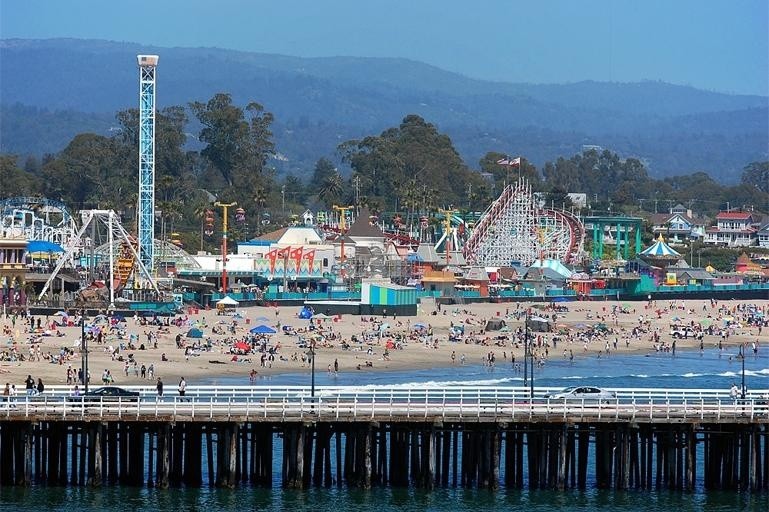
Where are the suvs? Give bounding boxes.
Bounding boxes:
[670,324,705,339]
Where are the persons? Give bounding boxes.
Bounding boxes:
[9,386,19,409]
[23,374,33,395]
[729,382,769,419]
[0,383,10,405]
[30,386,39,396]
[178,377,190,403]
[36,377,45,394]
[154,377,163,395]
[74,384,80,396]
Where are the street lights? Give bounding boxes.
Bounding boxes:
[80,307,90,391]
[736,344,747,418]
[306,338,315,414]
[78,336,89,413]
[524,315,532,404]
[526,342,535,416]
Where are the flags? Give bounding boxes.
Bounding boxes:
[496,156,509,165]
[509,158,520,168]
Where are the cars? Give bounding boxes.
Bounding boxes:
[544,385,623,409]
[68,386,142,408]
